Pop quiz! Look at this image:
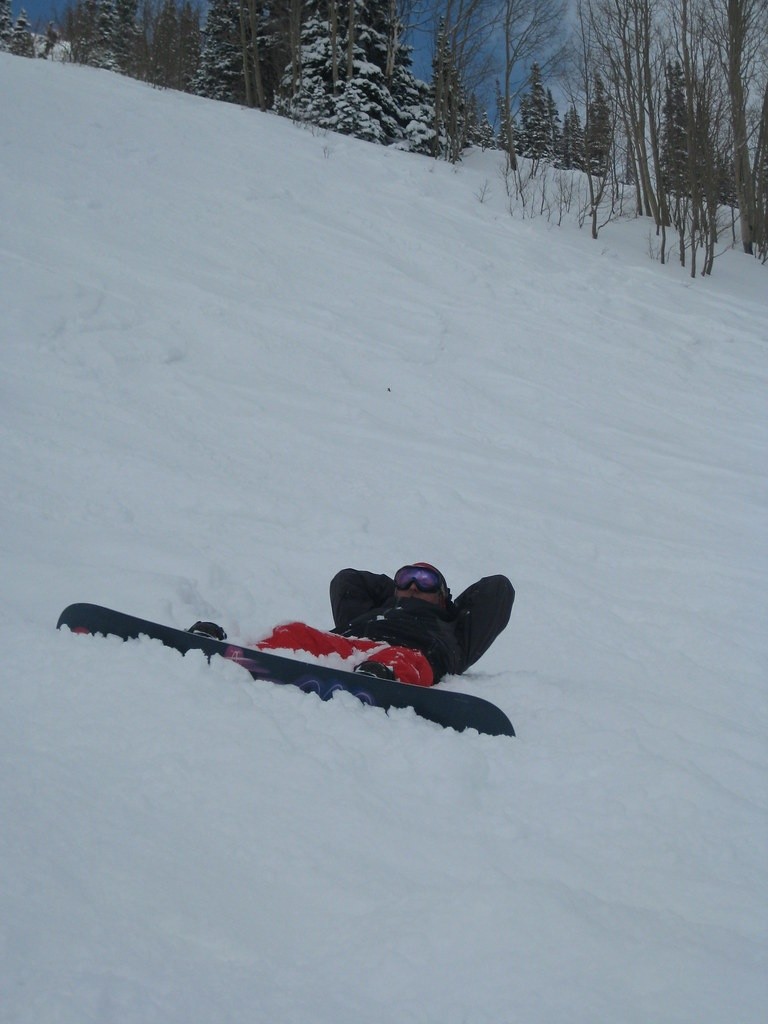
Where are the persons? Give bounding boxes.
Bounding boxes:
[187,563,515,686]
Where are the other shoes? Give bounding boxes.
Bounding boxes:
[185,619,228,642]
[351,659,396,684]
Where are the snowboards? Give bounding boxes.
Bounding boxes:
[55,602,514,738]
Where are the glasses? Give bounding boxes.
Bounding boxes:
[392,564,442,593]
